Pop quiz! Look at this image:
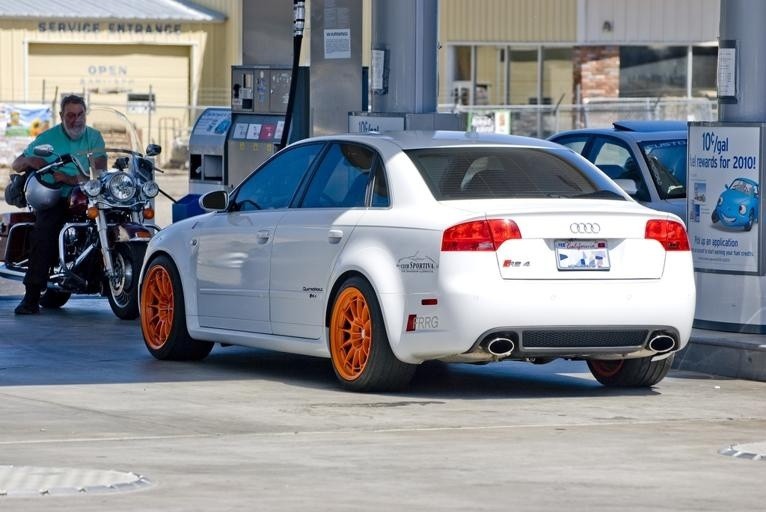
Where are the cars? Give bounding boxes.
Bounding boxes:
[542,119,689,220]
[137,130,696,395]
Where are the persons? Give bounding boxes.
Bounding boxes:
[10,96,108,315]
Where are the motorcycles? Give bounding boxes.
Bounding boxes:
[0,142,162,318]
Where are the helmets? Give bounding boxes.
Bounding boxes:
[24,171,63,211]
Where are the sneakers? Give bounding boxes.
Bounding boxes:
[14,299,40,315]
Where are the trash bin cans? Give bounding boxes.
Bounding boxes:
[187,103,234,197]
